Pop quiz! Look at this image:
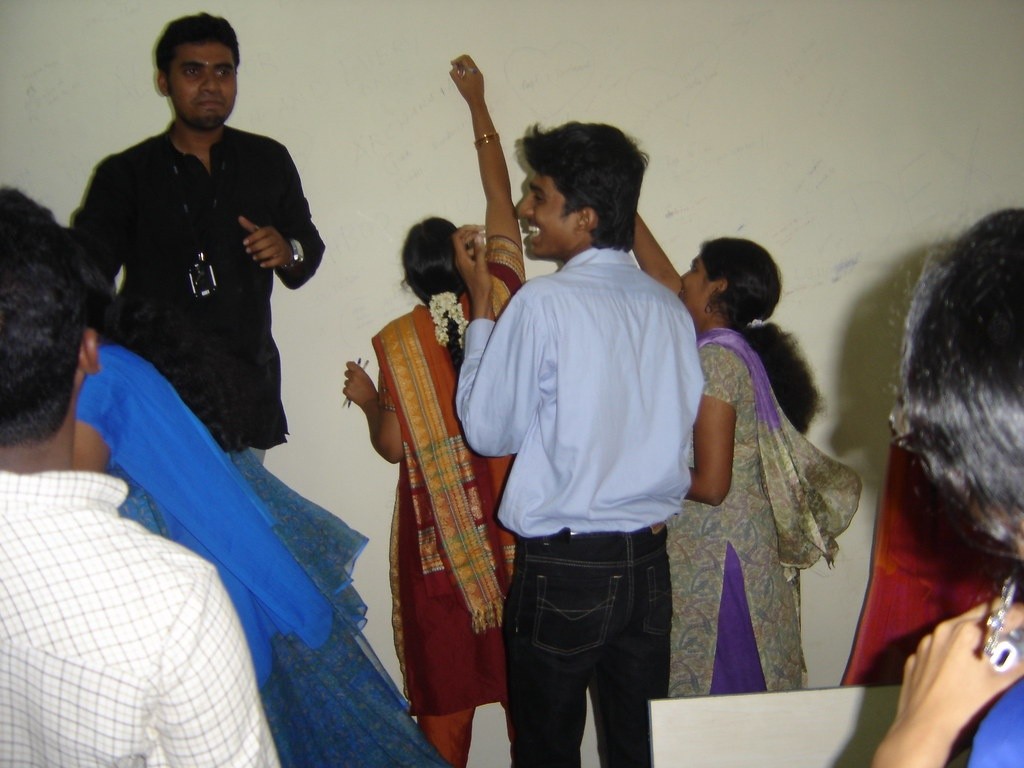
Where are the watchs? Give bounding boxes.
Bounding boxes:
[281,237,305,271]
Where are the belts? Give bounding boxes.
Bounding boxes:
[569,528,651,540]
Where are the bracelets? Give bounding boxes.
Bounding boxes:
[473,133,501,150]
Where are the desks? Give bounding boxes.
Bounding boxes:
[468,684,904,768]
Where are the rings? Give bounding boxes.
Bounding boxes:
[468,67,478,73]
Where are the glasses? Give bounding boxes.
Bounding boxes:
[888,401,919,454]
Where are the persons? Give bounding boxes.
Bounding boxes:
[0,186,455,768]
[869,205,1024,768]
[71,10,324,467]
[342,54,862,768]
